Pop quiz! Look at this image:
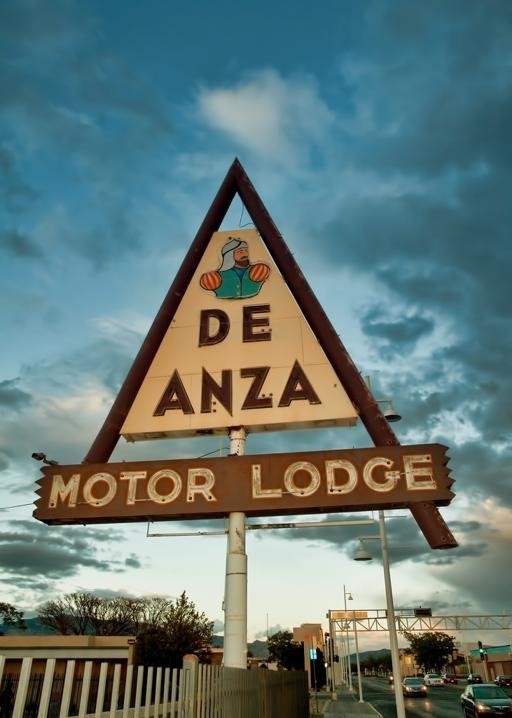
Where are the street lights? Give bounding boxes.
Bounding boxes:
[349,534,408,714]
[343,584,355,693]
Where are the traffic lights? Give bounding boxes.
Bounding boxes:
[479,647,487,655]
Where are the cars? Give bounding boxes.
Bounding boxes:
[459,683,512,718]
[386,671,512,693]
